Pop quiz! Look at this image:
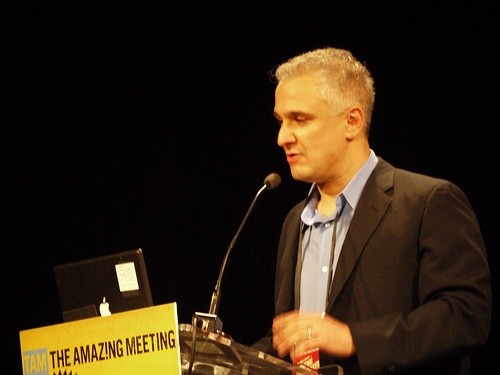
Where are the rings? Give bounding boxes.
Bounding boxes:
[320,311,326,320]
[308,328,312,338]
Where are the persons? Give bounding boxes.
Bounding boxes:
[246,46,492,375]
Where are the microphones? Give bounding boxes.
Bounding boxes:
[192,173,281,334]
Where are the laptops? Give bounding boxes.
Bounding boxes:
[54,248,153,322]
